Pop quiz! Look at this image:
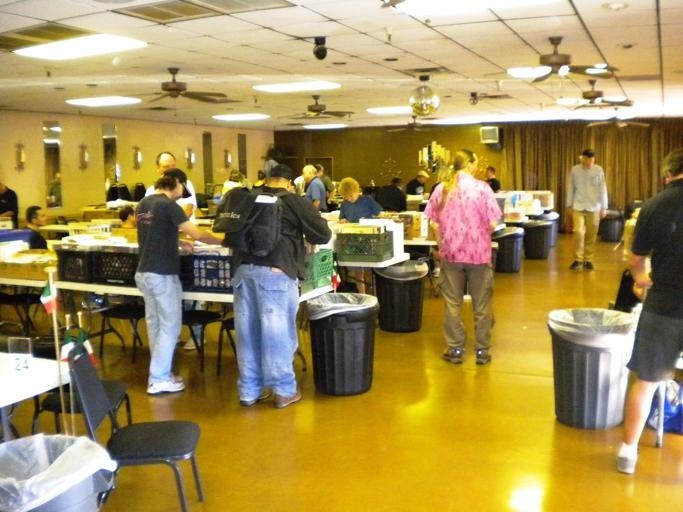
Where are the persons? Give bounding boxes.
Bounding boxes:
[485,166,499,193]
[225,162,334,411]
[221,160,265,199]
[613,145,682,475]
[132,166,225,395]
[290,165,452,294]
[564,148,610,273]
[144,152,206,349]
[0,181,18,231]
[119,206,137,227]
[423,148,504,366]
[20,206,106,311]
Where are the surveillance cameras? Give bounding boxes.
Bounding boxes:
[313,37,327,59]
[470,92,478,105]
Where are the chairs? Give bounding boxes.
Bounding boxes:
[68,344,203,512]
[209,184,223,199]
[106,183,146,202]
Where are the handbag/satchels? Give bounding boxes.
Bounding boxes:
[212,187,260,232]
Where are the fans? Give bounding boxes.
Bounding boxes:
[587,108,650,129]
[574,79,633,109]
[276,95,354,120]
[531,36,620,84]
[119,67,227,104]
[386,115,423,132]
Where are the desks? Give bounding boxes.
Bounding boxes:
[1,276,337,374]
[0,351,79,440]
[1,196,553,327]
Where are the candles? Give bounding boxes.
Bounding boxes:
[418,141,450,171]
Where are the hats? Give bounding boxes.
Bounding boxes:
[269,164,296,186]
[163,168,192,197]
[582,148,594,157]
[417,169,430,178]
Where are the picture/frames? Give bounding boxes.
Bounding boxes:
[303,156,334,181]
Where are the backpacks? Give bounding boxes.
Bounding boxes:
[221,185,292,258]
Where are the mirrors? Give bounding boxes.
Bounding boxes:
[237,133,247,179]
[102,123,121,202]
[202,131,213,194]
[40,120,63,208]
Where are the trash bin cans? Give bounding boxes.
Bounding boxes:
[601,209,625,242]
[305,293,381,395]
[531,209,561,248]
[491,226,525,273]
[522,219,553,259]
[546,307,639,430]
[0,432,120,512]
[373,260,429,333]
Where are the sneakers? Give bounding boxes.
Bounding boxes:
[146,372,185,395]
[274,390,303,408]
[240,388,271,406]
[583,261,594,270]
[442,346,462,364]
[475,349,490,364]
[569,261,583,270]
[433,271,440,277]
[617,456,637,473]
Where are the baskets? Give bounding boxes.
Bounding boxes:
[337,230,394,262]
[55,249,334,298]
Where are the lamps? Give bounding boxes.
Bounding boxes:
[187,148,195,170]
[132,144,141,170]
[78,143,88,172]
[224,149,231,168]
[14,142,25,175]
